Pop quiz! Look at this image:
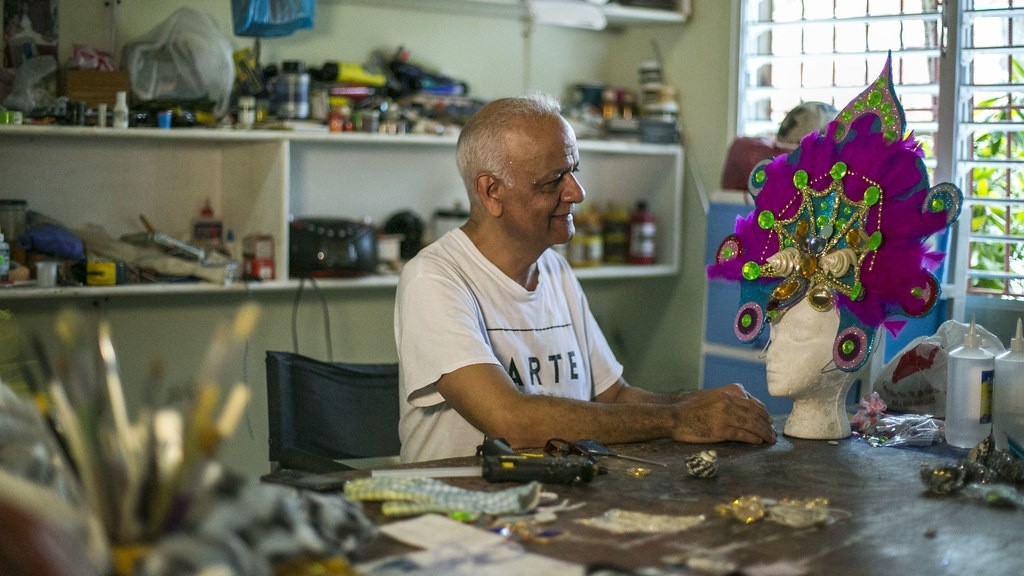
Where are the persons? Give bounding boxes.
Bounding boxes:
[393,94,778,463]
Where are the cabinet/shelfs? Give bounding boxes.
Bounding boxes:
[696,190,973,401]
[0,125,686,298]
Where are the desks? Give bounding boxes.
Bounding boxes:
[270,430,1023,576]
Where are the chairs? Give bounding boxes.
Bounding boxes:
[264,351,400,475]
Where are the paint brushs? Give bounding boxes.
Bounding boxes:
[3,295,265,544]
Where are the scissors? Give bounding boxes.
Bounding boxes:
[574,439,668,468]
